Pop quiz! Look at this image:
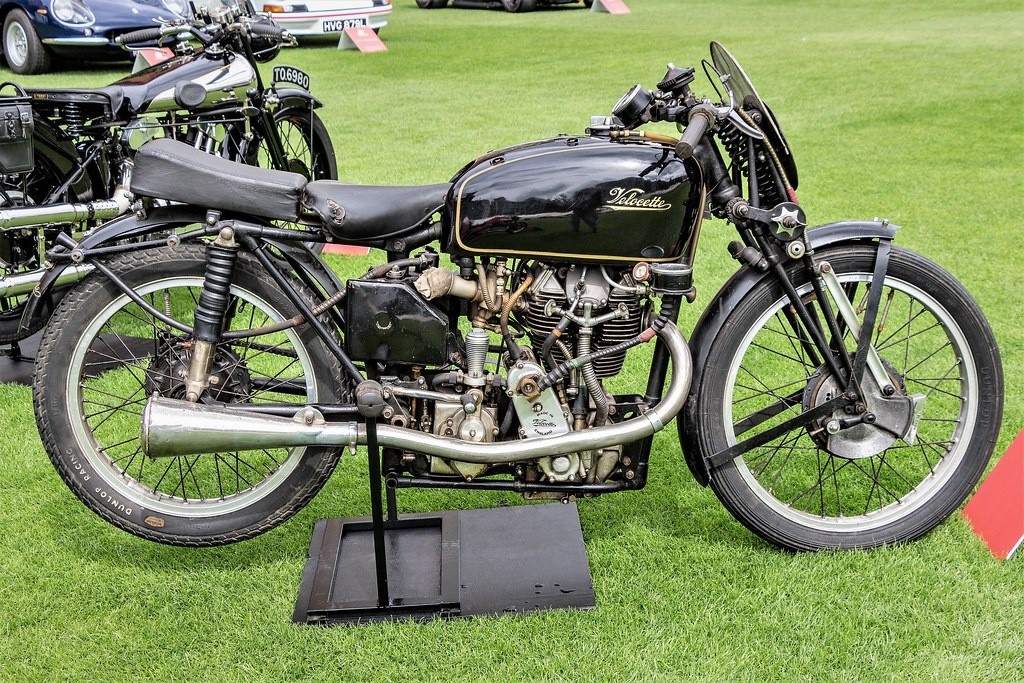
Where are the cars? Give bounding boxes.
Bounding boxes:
[0,0,393,76]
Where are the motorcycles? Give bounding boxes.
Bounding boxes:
[16,41,1005,553]
[0,0,339,346]
